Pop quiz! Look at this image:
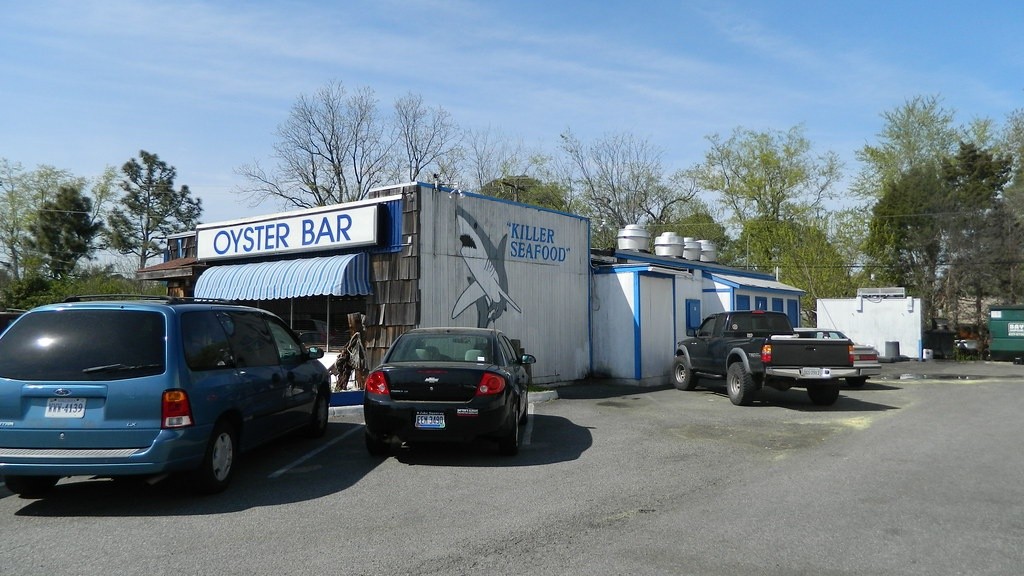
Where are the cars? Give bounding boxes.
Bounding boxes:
[794,327,883,387]
[362,327,537,457]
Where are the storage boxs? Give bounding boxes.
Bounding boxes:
[771,335,796,340]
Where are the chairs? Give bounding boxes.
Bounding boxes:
[465,350,484,361]
[410,349,430,360]
[311,319,327,332]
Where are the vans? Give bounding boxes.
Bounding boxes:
[0,294,331,503]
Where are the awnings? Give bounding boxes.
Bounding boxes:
[194,251,373,303]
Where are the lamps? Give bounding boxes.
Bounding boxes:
[434,177,467,200]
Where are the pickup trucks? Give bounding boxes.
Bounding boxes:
[670,309,855,407]
